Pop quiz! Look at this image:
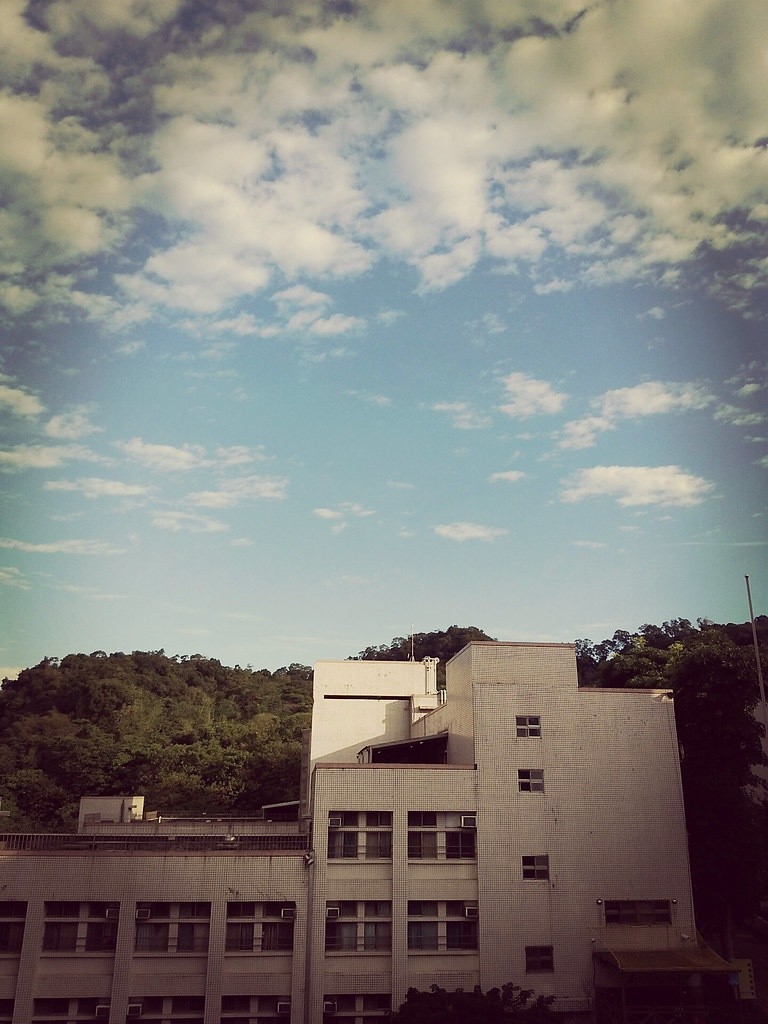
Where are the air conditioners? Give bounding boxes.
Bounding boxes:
[127,1004,143,1016]
[106,909,119,918]
[277,1002,291,1013]
[95,1005,110,1017]
[281,909,294,919]
[323,1002,338,1012]
[464,905,478,917]
[459,816,477,828]
[135,909,150,919]
[326,907,339,917]
[329,818,341,827]
